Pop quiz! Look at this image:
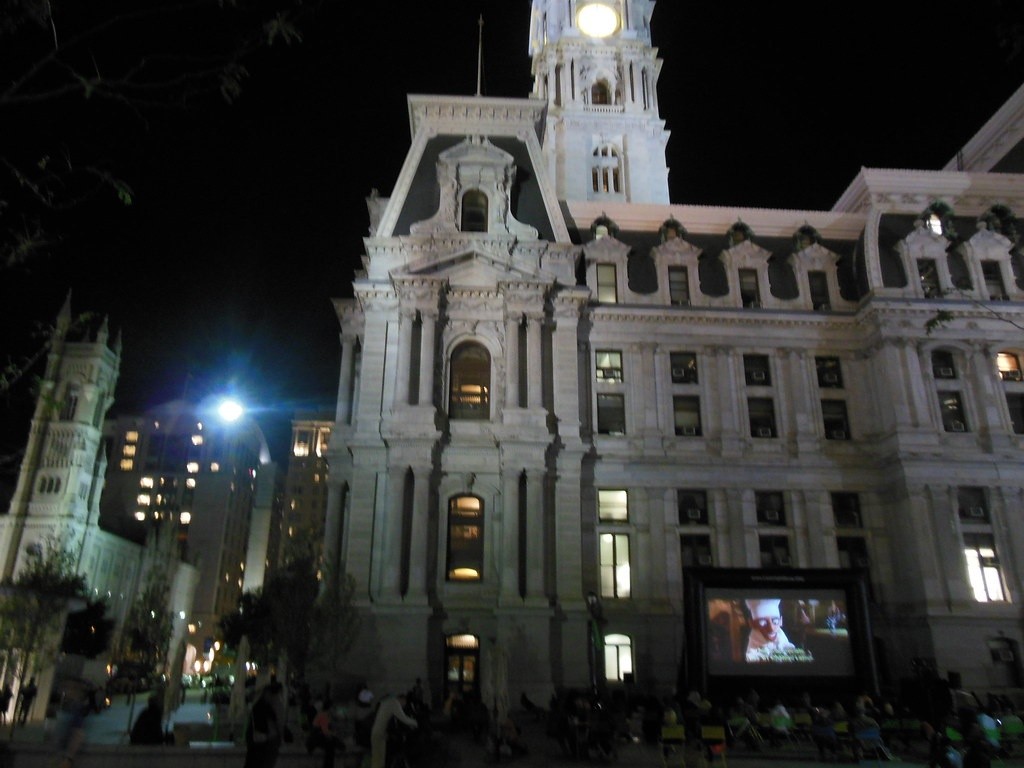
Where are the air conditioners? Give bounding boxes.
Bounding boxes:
[757,427,772,438]
[996,648,1016,662]
[950,421,965,432]
[698,554,713,566]
[752,371,765,383]
[1006,369,1021,379]
[938,367,953,377]
[672,367,684,377]
[823,373,838,383]
[752,301,760,309]
[689,508,701,519]
[679,298,691,306]
[682,425,696,436]
[966,506,984,520]
[856,558,872,568]
[765,510,779,522]
[832,430,846,440]
[777,555,792,566]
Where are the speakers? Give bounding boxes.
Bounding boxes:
[947,671,962,690]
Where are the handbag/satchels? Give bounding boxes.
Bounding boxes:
[252,732,267,743]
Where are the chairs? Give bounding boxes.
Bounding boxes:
[657,723,688,768]
[881,719,922,749]
[855,727,882,760]
[810,724,837,761]
[833,721,850,742]
[1001,714,1024,745]
[695,725,728,768]
[754,712,794,746]
[794,712,815,748]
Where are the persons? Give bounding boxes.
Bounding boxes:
[0,683,15,725]
[130,695,175,746]
[17,677,38,725]
[245,669,1024,768]
